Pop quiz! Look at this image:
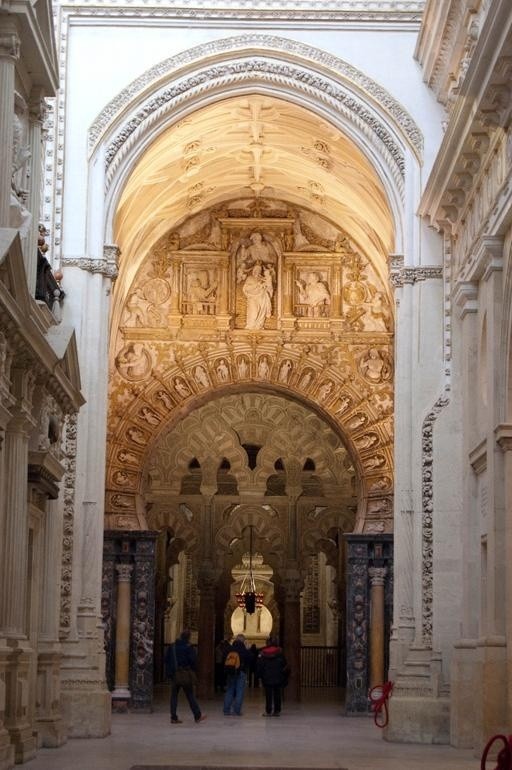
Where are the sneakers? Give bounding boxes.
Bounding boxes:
[195,713,207,724]
[171,718,183,724]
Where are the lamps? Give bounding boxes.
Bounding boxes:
[235,524,263,614]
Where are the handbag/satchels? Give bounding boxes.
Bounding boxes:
[173,670,200,688]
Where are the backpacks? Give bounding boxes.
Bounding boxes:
[224,650,242,681]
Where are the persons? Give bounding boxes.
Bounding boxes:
[114,358,209,529]
[215,634,258,716]
[360,348,391,383]
[162,630,207,724]
[359,278,389,332]
[293,272,330,305]
[258,640,292,717]
[123,287,148,328]
[279,360,388,532]
[237,358,248,378]
[118,344,147,376]
[217,360,227,380]
[259,357,269,378]
[187,278,217,299]
[236,229,279,327]
